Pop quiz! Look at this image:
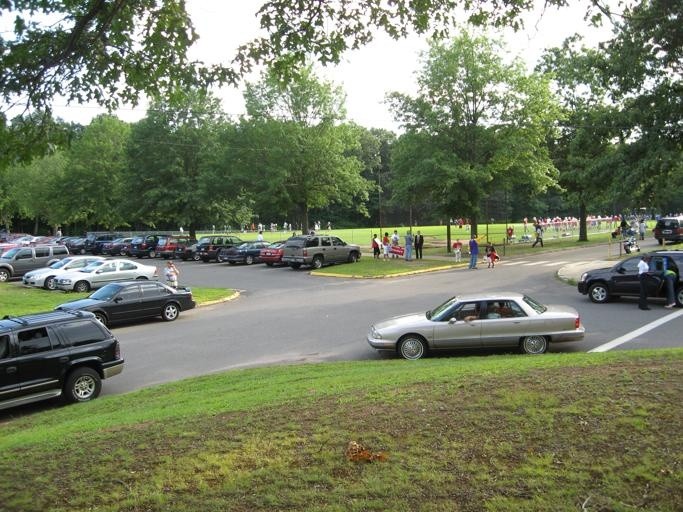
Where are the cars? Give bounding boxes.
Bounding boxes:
[365,293,584,361]
[57,259,159,293]
[22,255,106,290]
[53,281,196,329]
[0,233,285,267]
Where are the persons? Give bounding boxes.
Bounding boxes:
[504,210,681,249]
[495,302,510,317]
[257,222,265,241]
[328,222,332,232]
[180,226,184,235]
[652,268,678,310]
[212,221,255,234]
[269,222,292,234]
[452,234,497,269]
[487,304,500,319]
[372,230,424,261]
[636,252,655,311]
[464,302,480,322]
[163,260,179,289]
[313,221,321,232]
[450,216,472,232]
[55,226,64,244]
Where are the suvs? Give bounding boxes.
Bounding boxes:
[577,250,683,308]
[0,245,69,283]
[283,235,361,269]
[653,217,683,244]
[0,308,124,411]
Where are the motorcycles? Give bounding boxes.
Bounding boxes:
[622,236,640,253]
